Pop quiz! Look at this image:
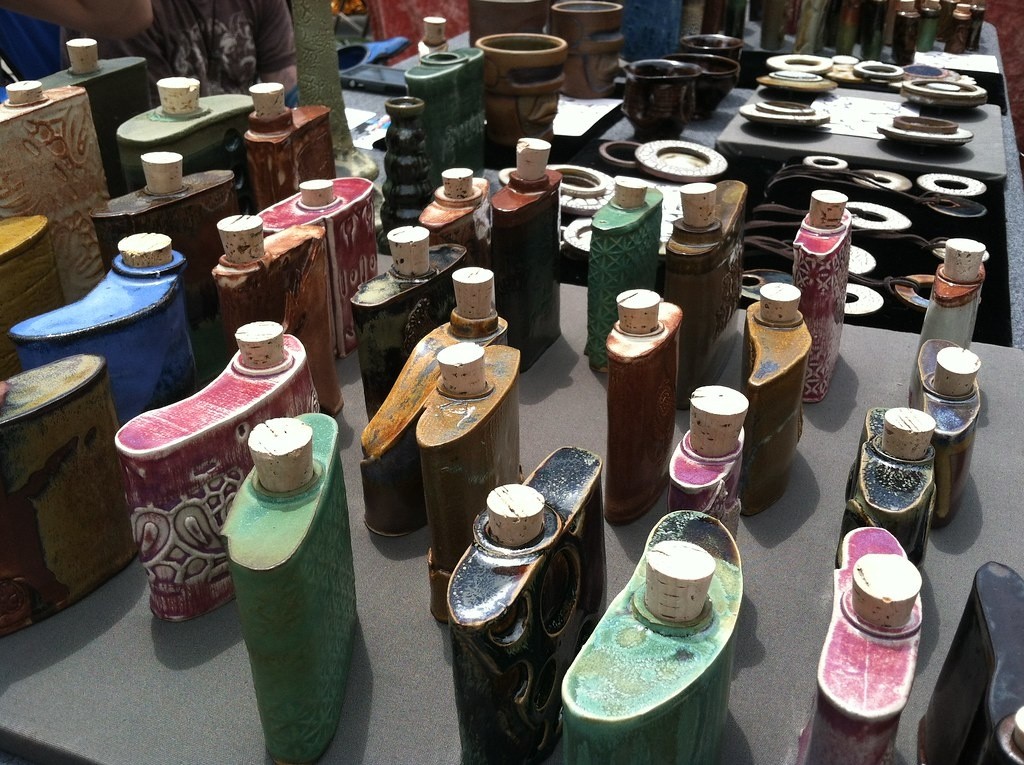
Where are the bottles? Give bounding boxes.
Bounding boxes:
[490,136,561,372]
[404,48,485,191]
[377,96,431,255]
[796,525,924,765]
[682,0,985,67]
[908,338,980,529]
[116,76,254,216]
[242,80,335,211]
[0,150,853,764]
[0,79,111,306]
[833,407,938,571]
[39,37,156,198]
[908,239,988,398]
[924,561,1024,765]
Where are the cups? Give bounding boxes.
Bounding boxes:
[477,33,567,144]
[551,0,623,101]
[623,59,703,144]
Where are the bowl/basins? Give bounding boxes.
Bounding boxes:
[662,35,745,120]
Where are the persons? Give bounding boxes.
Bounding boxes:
[0,0,300,107]
